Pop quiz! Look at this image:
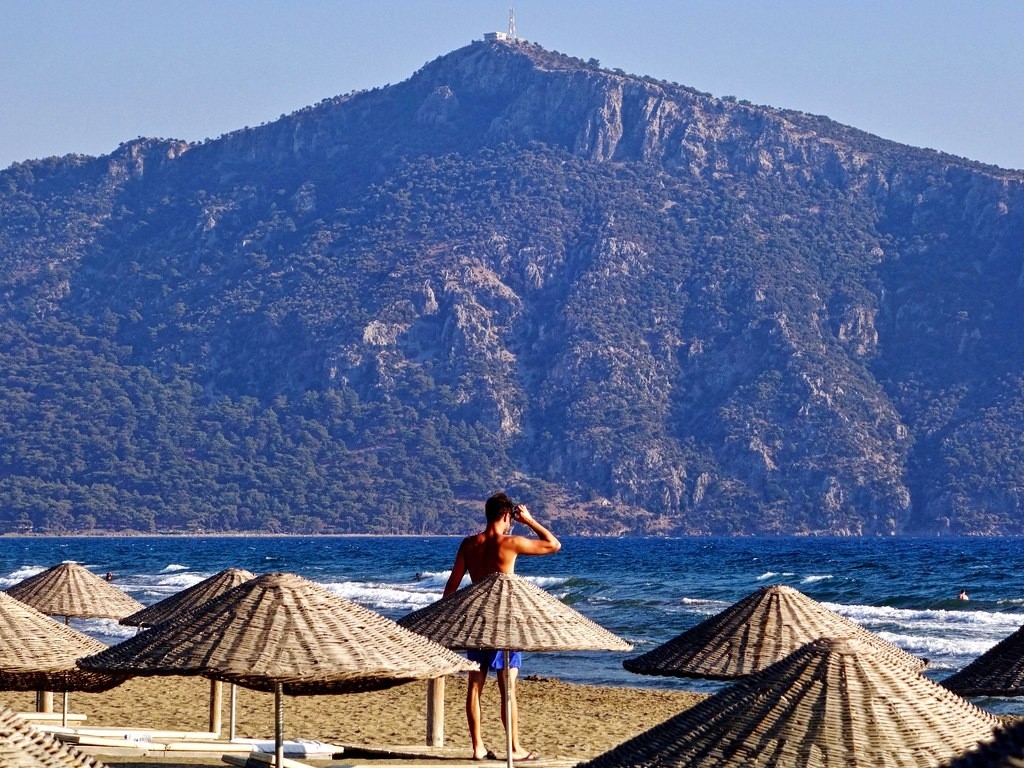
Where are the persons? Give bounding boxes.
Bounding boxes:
[441,491,563,763]
[105,572,112,581]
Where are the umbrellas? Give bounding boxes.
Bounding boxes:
[623,583,934,685]
[395,570,636,768]
[570,631,1024,768]
[0,588,112,676]
[119,566,258,746]
[75,572,484,768]
[937,622,1024,699]
[0,561,150,726]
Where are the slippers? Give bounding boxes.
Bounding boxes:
[513,751,540,761]
[472,746,497,760]
